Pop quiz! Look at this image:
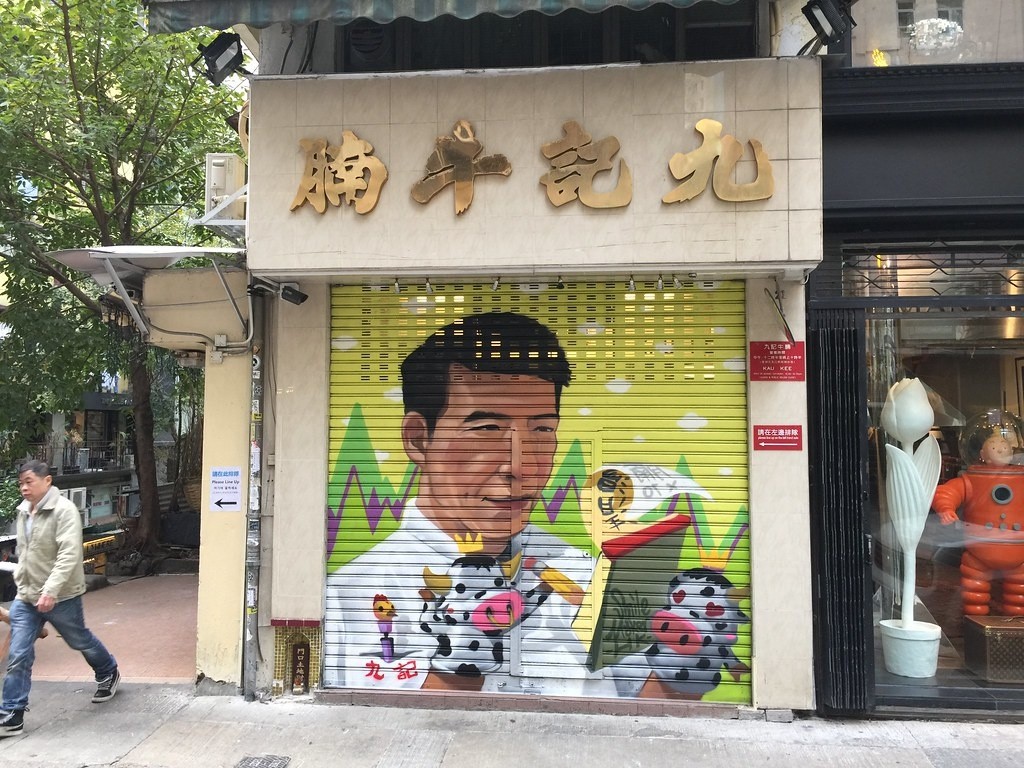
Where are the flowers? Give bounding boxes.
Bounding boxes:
[881,378,942,628]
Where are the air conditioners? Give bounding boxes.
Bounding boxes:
[205,153,246,220]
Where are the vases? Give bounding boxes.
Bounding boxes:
[879,620,942,679]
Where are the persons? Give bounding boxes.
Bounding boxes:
[0,457,121,738]
[932,424,1024,616]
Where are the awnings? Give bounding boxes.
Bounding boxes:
[143,0,743,34]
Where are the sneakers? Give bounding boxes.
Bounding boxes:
[0,707,30,736]
[92,669,122,701]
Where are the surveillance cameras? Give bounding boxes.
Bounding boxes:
[281,286,308,305]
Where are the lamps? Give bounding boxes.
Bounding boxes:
[190,32,253,86]
[224,111,249,135]
[801,0,858,45]
